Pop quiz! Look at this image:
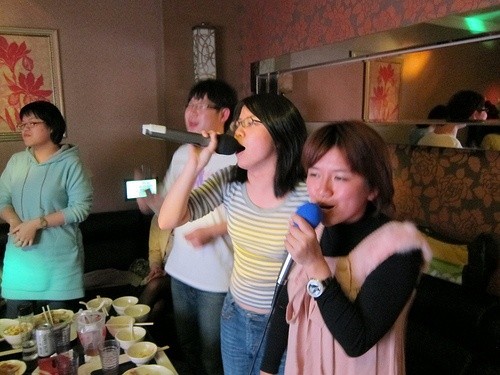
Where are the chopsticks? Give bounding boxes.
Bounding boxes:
[42,304,55,327]
[105,322,153,326]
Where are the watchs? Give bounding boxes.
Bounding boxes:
[307,276,333,298]
[40,217,47,230]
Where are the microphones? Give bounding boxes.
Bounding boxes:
[268,203,322,307]
[142,123,239,155]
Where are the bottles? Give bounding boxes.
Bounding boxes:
[17,304,38,361]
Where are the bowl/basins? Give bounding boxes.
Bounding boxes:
[85,295,174,375]
[1,322,33,350]
[35,308,74,324]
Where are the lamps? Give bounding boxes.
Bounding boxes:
[192,21,216,83]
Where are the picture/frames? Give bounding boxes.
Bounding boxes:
[0,26,67,142]
[362,57,403,122]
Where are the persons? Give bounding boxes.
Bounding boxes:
[410,90,500,150]
[134,78,237,375]
[0,101,94,318]
[158,93,309,375]
[260,120,431,375]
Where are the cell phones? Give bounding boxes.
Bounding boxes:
[125,178,158,200]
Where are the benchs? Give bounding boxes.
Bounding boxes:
[0,207,154,297]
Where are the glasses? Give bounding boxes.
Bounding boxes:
[476,106,491,115]
[16,120,45,131]
[184,101,220,113]
[235,116,263,128]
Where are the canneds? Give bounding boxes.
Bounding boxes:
[36,323,55,358]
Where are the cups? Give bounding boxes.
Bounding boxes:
[97,339,121,375]
[70,310,106,356]
[53,323,79,375]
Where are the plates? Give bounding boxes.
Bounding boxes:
[0,359,27,375]
[0,318,18,341]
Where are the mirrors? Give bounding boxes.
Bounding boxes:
[250,5,500,152]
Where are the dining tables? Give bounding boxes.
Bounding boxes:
[0,284,206,375]
